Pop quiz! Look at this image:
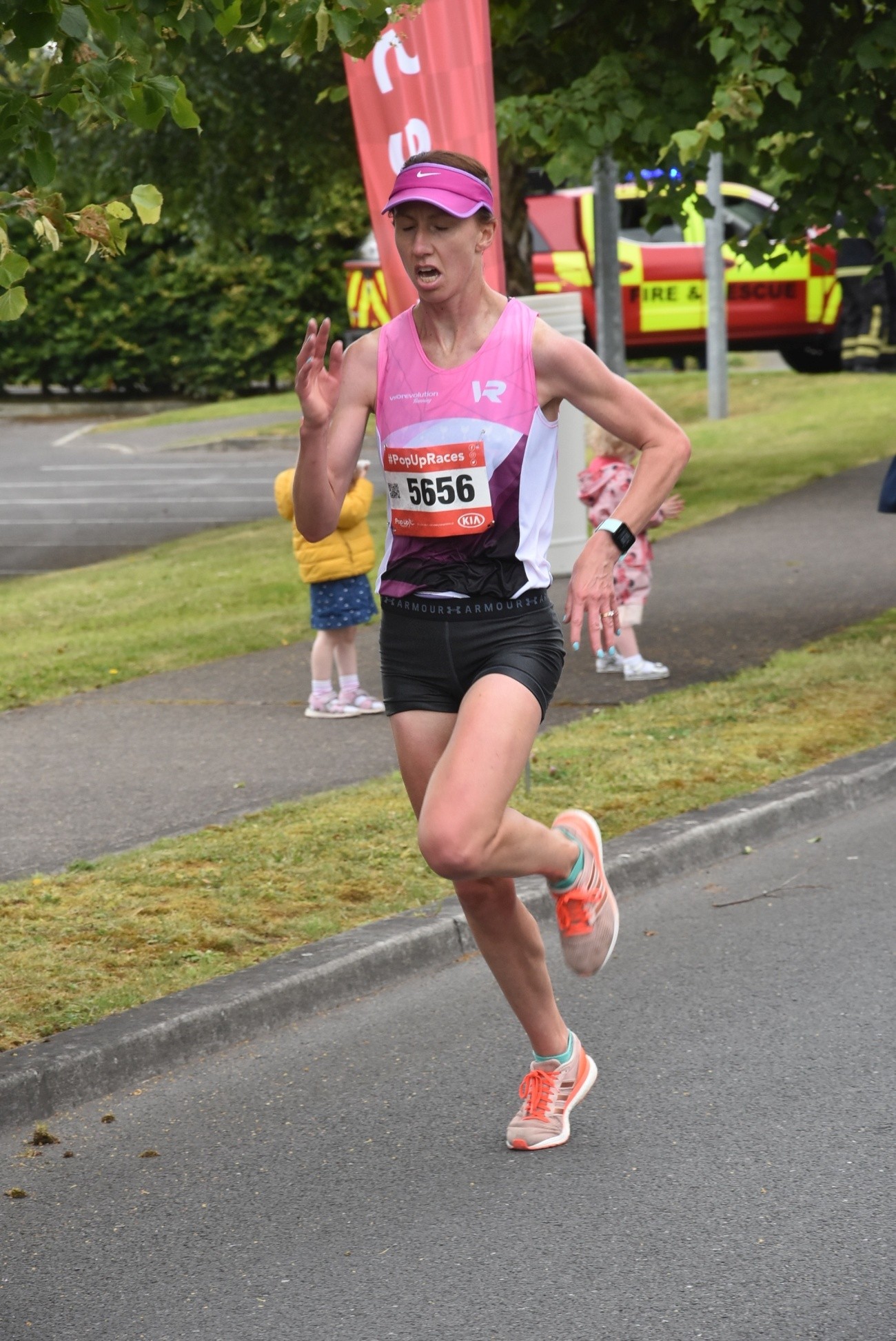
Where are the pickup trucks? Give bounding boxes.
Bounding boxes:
[336,163,847,374]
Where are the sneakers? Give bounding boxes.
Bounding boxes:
[544,810,619,978]
[504,1032,599,1149]
[624,660,669,680]
[596,653,627,674]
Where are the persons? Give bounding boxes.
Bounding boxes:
[576,422,686,681]
[293,149,692,1150]
[273,416,385,718]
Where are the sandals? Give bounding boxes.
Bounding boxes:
[303,691,361,719]
[337,687,384,716]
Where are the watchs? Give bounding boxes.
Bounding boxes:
[594,516,636,555]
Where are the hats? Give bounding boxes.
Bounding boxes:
[379,165,495,224]
